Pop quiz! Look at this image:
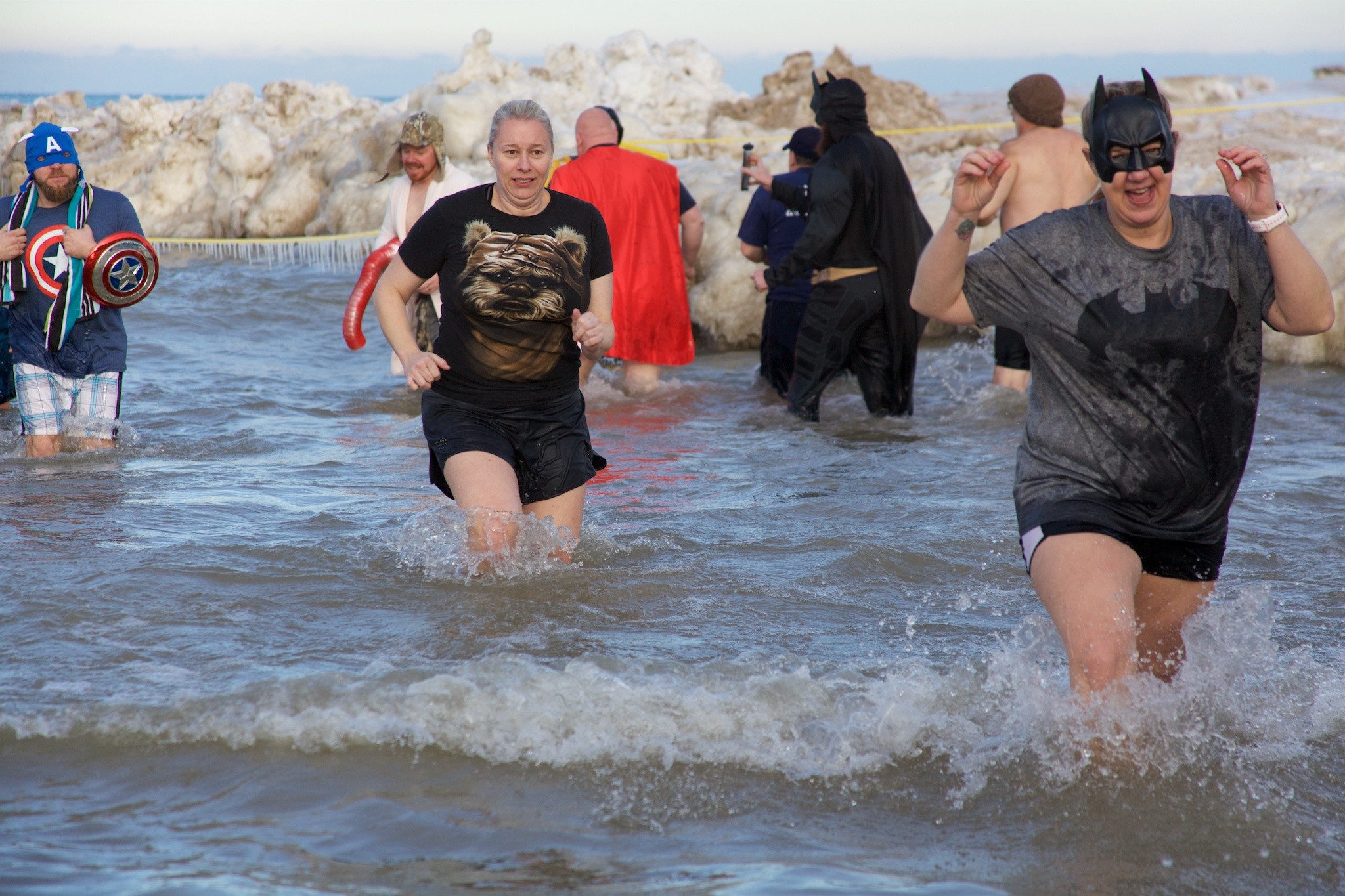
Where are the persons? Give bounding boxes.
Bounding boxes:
[736,127,822,399]
[373,99,615,573]
[740,70,934,419]
[376,111,483,376]
[549,107,707,392]
[909,67,1336,707]
[0,122,146,457]
[976,74,1099,392]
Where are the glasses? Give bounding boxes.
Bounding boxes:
[1008,102,1014,111]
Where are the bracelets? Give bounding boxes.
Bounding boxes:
[1247,200,1289,233]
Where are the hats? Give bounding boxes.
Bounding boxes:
[1009,75,1065,127]
[17,122,86,192]
[783,127,820,158]
[386,111,446,182]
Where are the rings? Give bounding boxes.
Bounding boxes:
[1266,166,1271,172]
[1262,154,1268,160]
